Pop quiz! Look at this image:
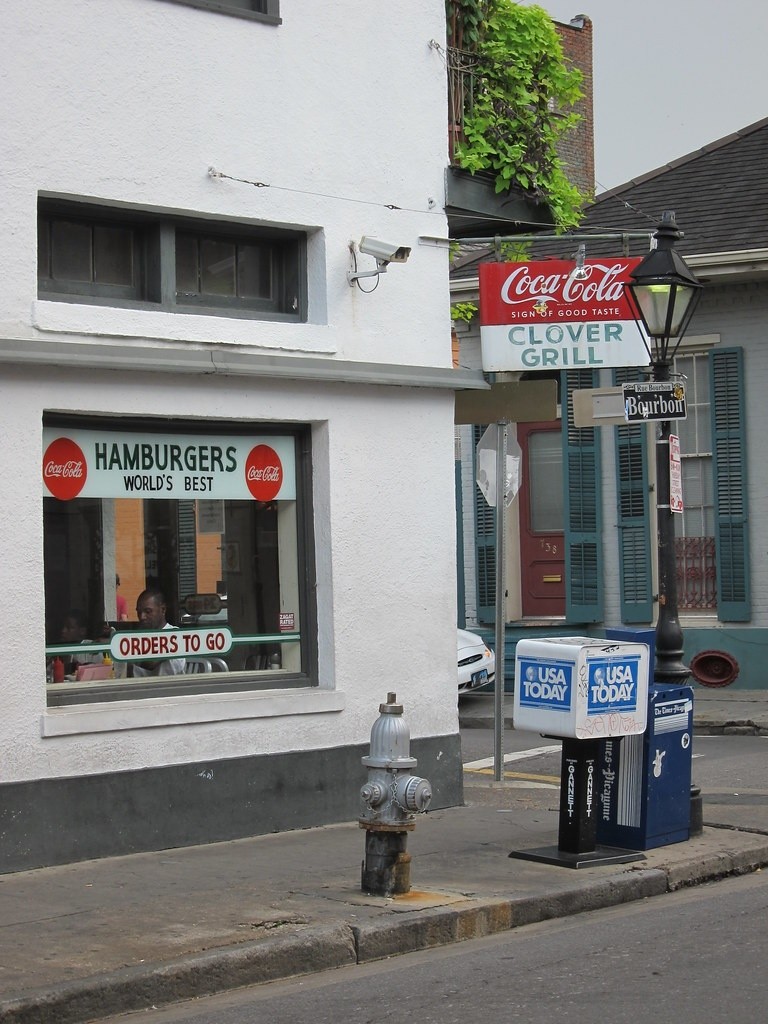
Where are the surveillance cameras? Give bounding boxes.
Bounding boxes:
[358,235,412,263]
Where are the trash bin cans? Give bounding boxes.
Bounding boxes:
[596,626,694,852]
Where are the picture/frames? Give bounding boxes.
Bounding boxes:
[220,541,242,574]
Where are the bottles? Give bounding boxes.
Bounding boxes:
[103,653,112,666]
[54,655,64,683]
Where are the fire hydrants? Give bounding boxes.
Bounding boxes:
[356,691,432,896]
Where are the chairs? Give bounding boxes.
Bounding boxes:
[178,656,231,675]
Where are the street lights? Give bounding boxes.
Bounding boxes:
[623,212,705,834]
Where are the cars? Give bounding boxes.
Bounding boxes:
[457,629,497,701]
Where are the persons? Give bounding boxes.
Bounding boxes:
[46,610,105,666]
[107,589,185,678]
[116,573,129,622]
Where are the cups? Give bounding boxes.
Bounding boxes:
[113,662,127,678]
